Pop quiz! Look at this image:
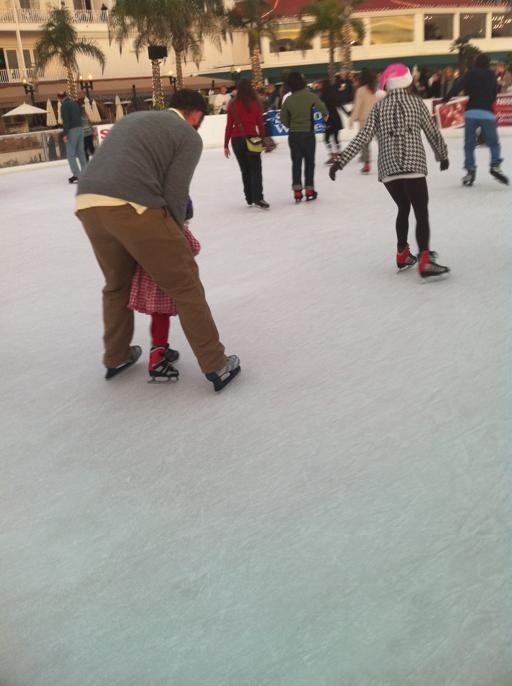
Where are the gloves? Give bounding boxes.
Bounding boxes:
[440,159,450,171]
[329,163,341,181]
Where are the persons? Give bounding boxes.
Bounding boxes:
[495,63,511,93]
[224,79,270,210]
[412,65,461,101]
[76,89,241,392]
[57,90,87,184]
[129,193,200,385]
[255,81,285,114]
[74,100,95,164]
[319,81,344,163]
[280,71,329,203]
[437,53,509,187]
[330,63,451,283]
[330,65,355,143]
[215,86,237,115]
[348,67,386,173]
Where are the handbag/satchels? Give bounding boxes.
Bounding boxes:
[230,101,263,152]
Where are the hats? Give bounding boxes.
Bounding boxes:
[381,64,413,91]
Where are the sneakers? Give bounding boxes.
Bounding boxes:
[490,166,508,182]
[247,198,254,204]
[462,169,474,181]
[361,162,369,171]
[326,154,337,164]
[417,250,448,276]
[148,348,178,376]
[206,355,239,381]
[104,345,142,369]
[305,189,317,196]
[164,344,178,361]
[254,199,269,207]
[295,191,302,198]
[396,245,418,267]
[69,176,80,181]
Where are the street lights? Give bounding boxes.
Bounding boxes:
[77,73,94,105]
[23,77,35,106]
